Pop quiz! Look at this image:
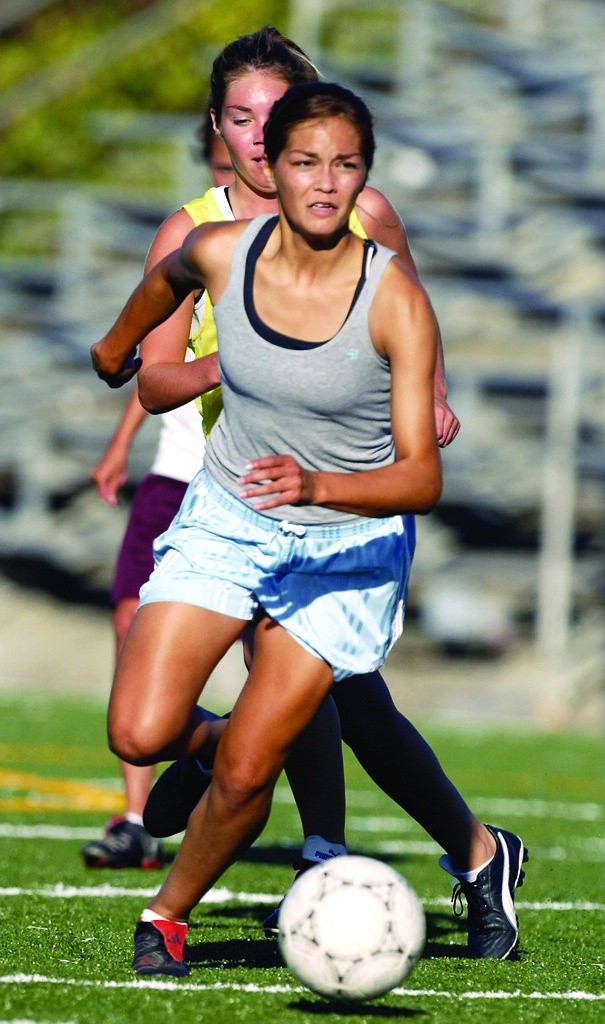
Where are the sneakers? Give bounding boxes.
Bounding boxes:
[438,824,529,961]
[80,815,162,870]
[263,858,321,938]
[143,711,232,838]
[131,919,191,977]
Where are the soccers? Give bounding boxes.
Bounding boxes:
[277,853,429,1005]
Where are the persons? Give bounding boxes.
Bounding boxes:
[134,27,530,961]
[81,116,237,863]
[94,78,442,972]
